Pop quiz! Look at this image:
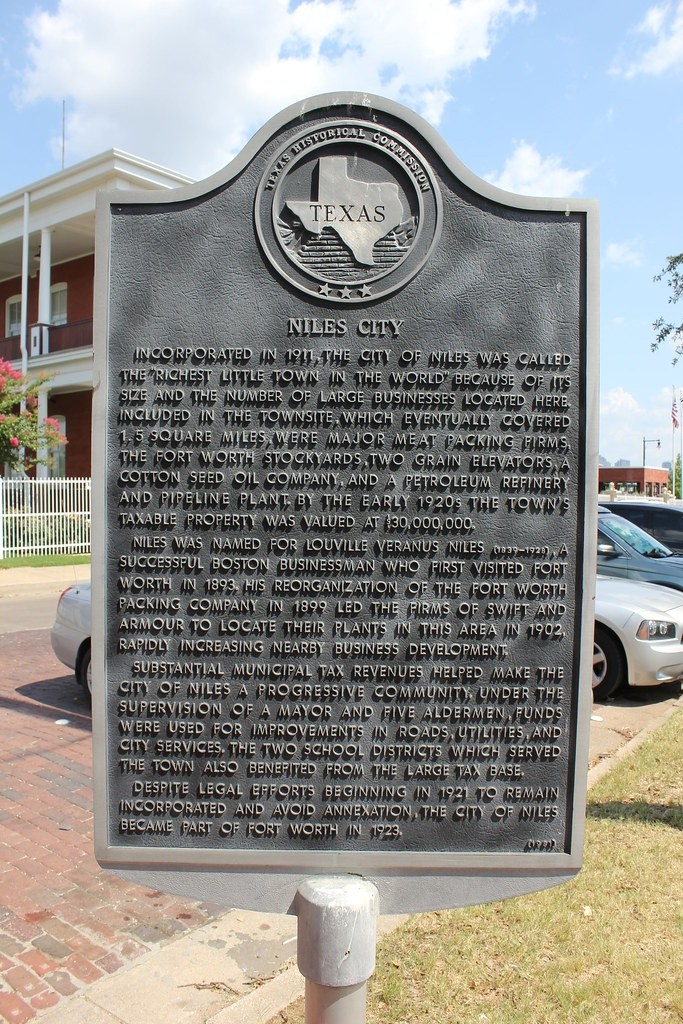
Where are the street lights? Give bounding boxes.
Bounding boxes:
[643,438,661,467]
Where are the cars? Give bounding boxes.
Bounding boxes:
[594,574,683,703]
[50,583,91,704]
[598,504,682,592]
[599,503,683,556]
